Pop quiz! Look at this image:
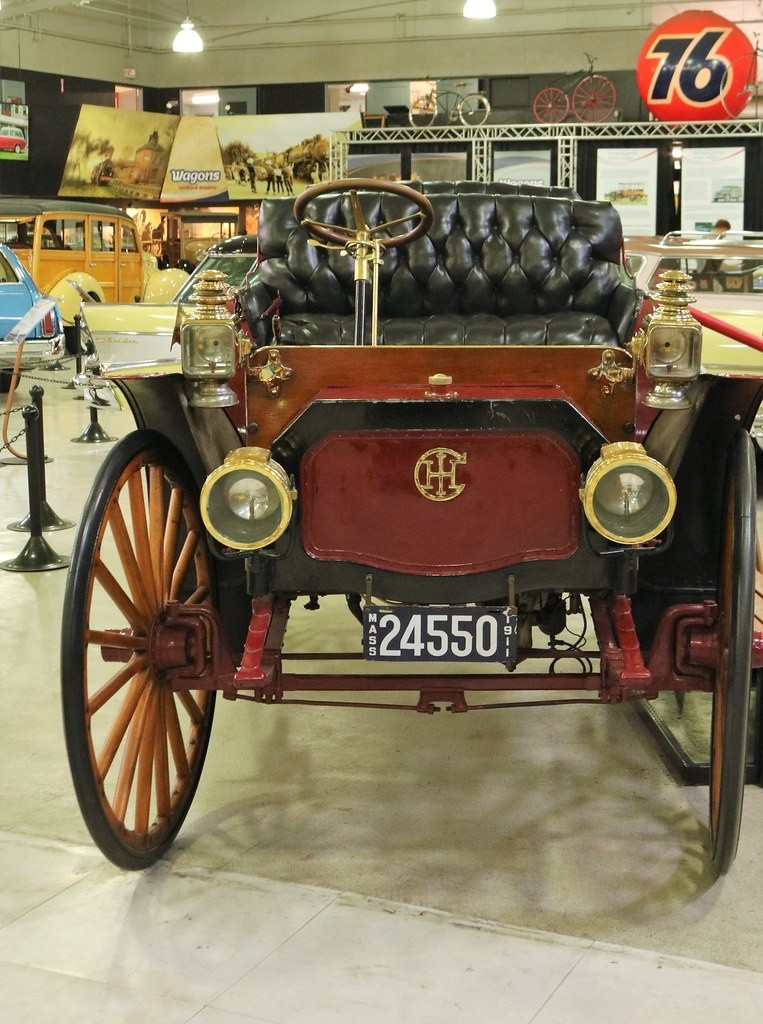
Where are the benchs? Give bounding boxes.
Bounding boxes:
[237,179,645,345]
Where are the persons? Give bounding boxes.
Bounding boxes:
[229,153,297,196]
[696,219,732,272]
[310,161,330,184]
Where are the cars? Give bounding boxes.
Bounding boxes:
[1,197,194,333]
[616,230,763,380]
[0,126,26,155]
[713,186,741,202]
[0,243,66,395]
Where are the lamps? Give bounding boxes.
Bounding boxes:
[225,104,234,115]
[165,101,179,113]
[172,0,204,52]
[463,0,496,19]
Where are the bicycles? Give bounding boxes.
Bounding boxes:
[408,74,492,127]
[533,50,617,124]
